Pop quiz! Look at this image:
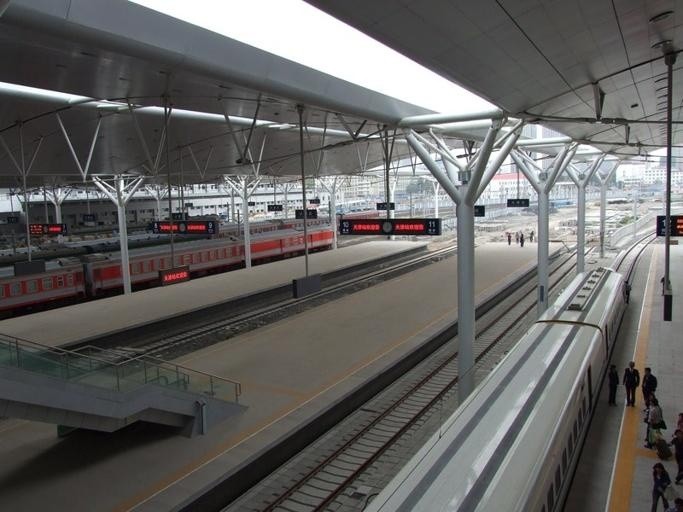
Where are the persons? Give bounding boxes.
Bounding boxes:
[659,274,671,296]
[506,230,534,247]
[607,357,683,512]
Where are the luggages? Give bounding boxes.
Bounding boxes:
[654,431,672,460]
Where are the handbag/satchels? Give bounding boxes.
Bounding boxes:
[664,485,678,500]
[653,421,666,429]
